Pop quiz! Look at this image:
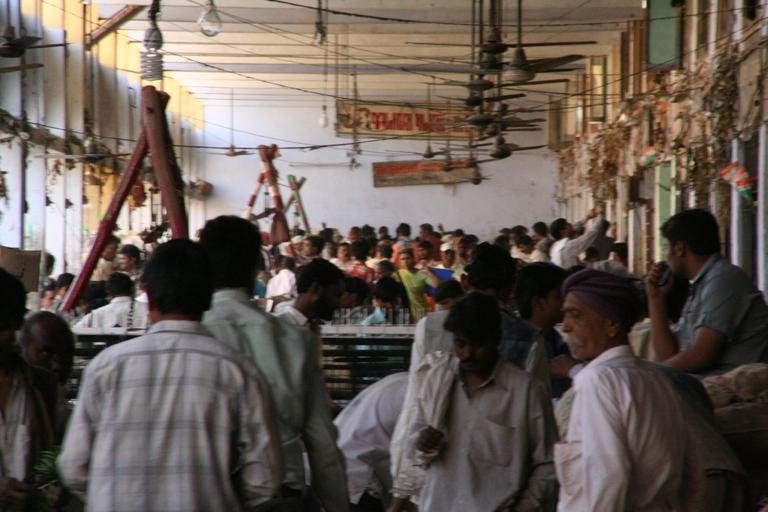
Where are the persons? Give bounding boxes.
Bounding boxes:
[0,201,768,512]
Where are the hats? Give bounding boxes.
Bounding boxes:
[440,243,454,253]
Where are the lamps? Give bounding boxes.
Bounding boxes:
[317,0,330,128]
[141,9,167,81]
[84,163,104,186]
[199,0,223,37]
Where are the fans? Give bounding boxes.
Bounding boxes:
[405,0,598,185]
[210,86,255,158]
[0,1,70,58]
